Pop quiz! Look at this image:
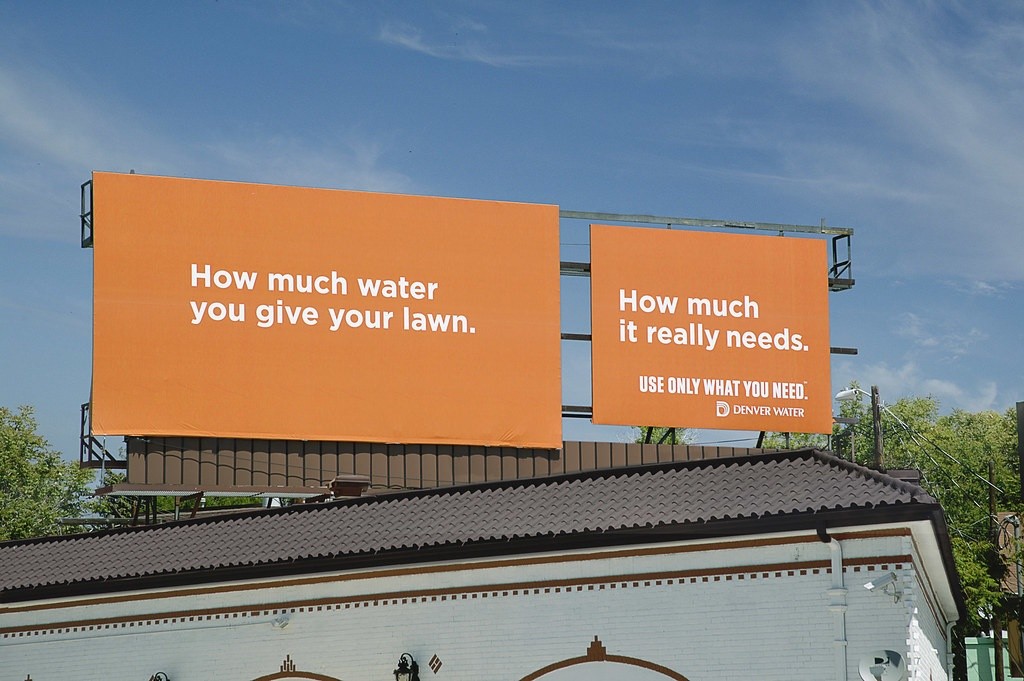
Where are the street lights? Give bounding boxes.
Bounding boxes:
[835,387,924,473]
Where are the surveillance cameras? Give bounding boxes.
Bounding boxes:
[864,572,897,592]
